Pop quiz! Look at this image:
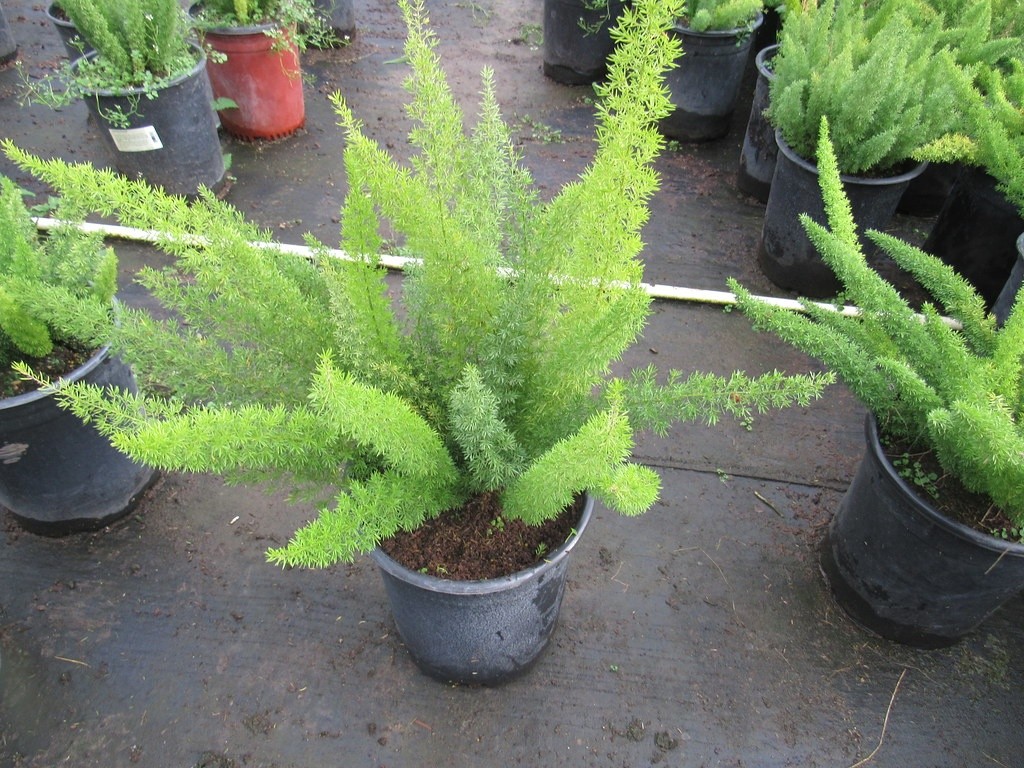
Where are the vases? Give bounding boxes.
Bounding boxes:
[544,0,632,87]
[43,1,95,65]
[0,3,18,65]
[297,1,357,49]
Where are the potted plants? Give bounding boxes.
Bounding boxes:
[0,173,159,535]
[722,113,1024,652]
[190,0,354,144]
[734,1,1024,329]
[655,0,764,145]
[13,1,229,209]
[10,1,838,691]
